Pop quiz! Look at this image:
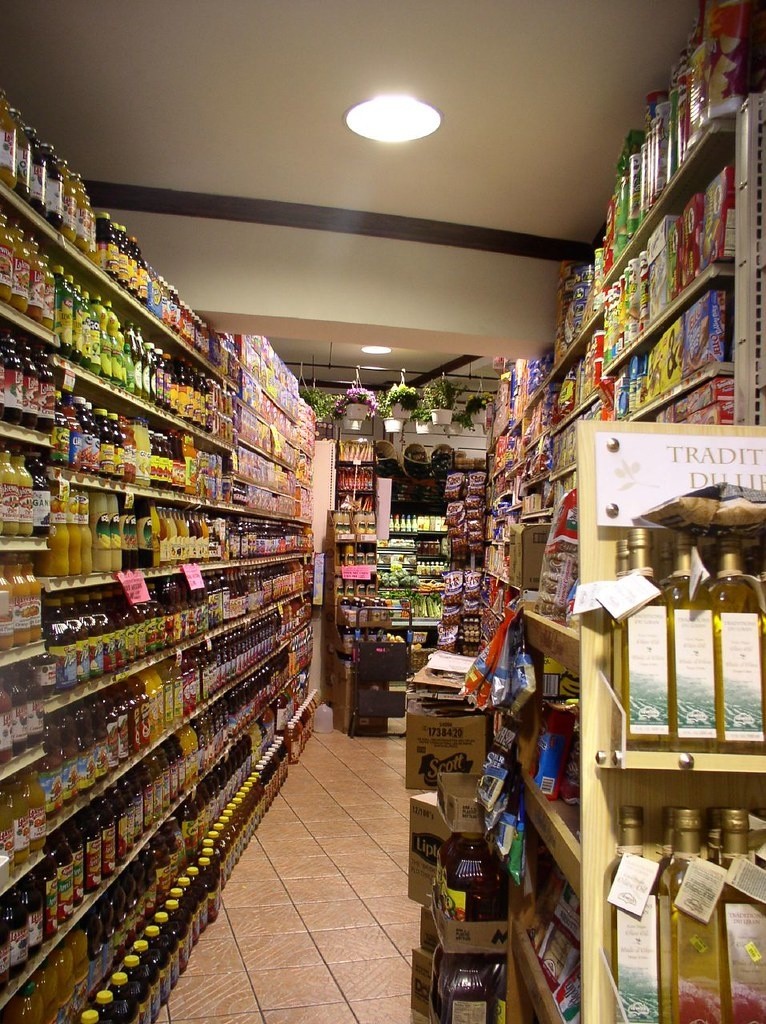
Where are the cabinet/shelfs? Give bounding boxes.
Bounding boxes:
[478,0,766,1024]
[321,440,484,738]
[0,92,315,1024]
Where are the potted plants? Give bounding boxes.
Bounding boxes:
[388,383,420,420]
[423,378,466,426]
[375,391,404,432]
[443,411,475,436]
[300,388,335,422]
[410,399,432,434]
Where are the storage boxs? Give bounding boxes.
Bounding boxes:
[403,656,504,1022]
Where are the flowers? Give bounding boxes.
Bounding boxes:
[467,391,495,412]
[337,388,376,418]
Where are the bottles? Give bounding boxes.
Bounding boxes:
[416,522,766,1024]
[3,95,335,1024]
[333,509,416,671]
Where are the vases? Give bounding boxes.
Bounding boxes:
[346,404,368,421]
[342,416,362,430]
[469,407,487,423]
